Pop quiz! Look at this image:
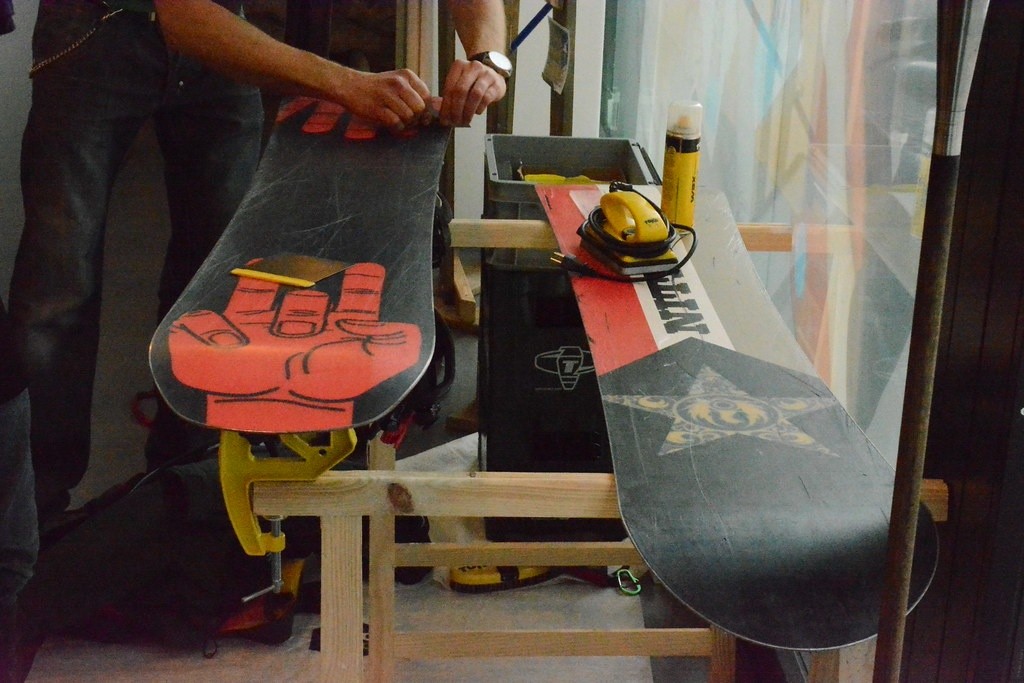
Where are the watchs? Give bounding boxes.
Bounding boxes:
[466,50,512,82]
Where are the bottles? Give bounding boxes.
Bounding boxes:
[657,98,704,233]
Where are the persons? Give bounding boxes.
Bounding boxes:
[0,264,59,681]
[4,1,512,541]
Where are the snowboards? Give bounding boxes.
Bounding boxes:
[148,84,459,440]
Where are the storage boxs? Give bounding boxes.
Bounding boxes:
[484,132,664,274]
